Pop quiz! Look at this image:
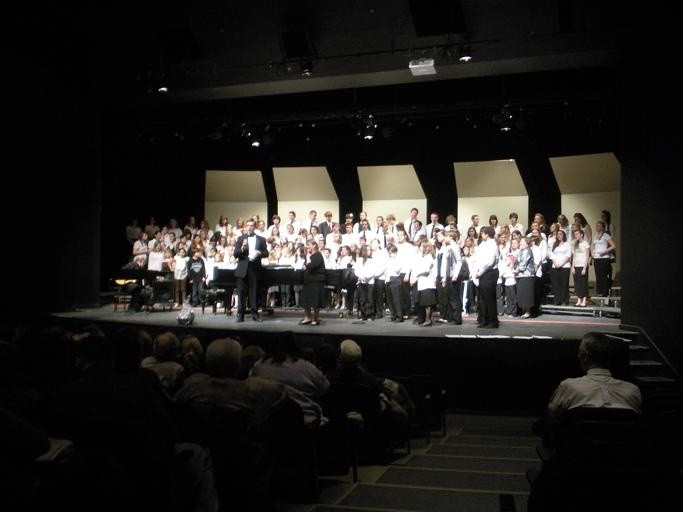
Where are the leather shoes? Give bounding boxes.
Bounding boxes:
[251,311,261,322]
[390,312,404,322]
[477,319,500,329]
[234,311,244,323]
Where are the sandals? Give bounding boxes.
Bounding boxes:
[299,316,320,326]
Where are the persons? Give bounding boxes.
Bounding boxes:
[121,207,617,330]
[0,318,416,512]
[545,332,644,415]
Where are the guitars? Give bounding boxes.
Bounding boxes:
[115,280,136,285]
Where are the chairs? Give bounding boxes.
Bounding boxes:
[525,383,683,512]
[130,372,447,512]
[111,267,344,317]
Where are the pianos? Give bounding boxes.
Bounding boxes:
[208,264,358,318]
[113,268,159,280]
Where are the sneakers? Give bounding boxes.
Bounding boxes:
[358,312,383,322]
[413,313,464,327]
[498,307,532,319]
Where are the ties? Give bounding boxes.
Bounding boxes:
[303,219,436,250]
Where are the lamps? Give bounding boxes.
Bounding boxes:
[239,101,521,153]
[152,39,473,93]
